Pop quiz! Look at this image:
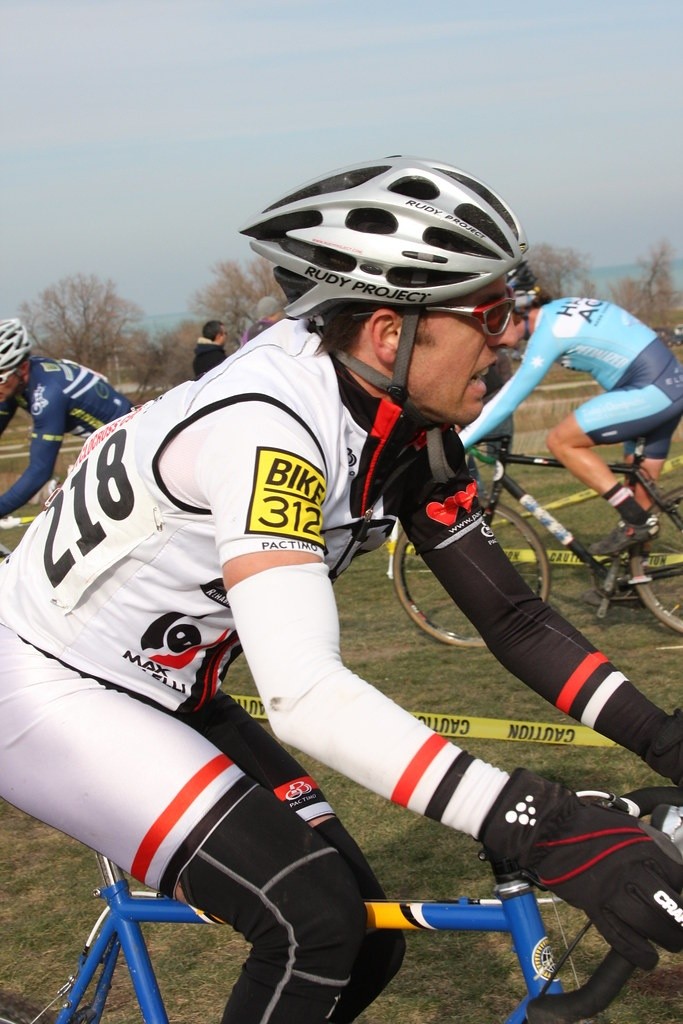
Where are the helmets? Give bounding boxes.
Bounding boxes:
[0,318,32,372]
[237,151,529,321]
[501,261,541,306]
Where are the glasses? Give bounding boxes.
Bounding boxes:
[0,366,18,384]
[350,285,516,337]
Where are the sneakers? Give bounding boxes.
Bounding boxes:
[587,514,660,559]
[596,579,649,599]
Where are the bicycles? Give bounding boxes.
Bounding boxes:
[393,431,683,649]
[58,733,683,1024]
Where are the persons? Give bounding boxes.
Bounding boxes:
[192,320,227,378]
[0,156,683,1024]
[458,257,683,555]
[482,349,513,463]
[0,318,135,521]
[240,296,282,346]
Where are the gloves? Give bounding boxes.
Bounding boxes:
[478,763,683,972]
[642,709,683,784]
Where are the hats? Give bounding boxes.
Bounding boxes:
[256,295,279,316]
